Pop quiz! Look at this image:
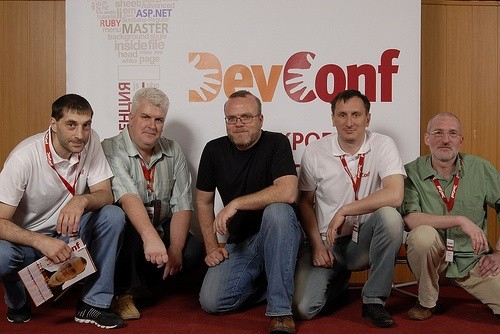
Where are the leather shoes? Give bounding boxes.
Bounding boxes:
[270,315,296,334]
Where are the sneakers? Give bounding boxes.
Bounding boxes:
[362,304,393,326]
[112,295,140,319]
[75,301,124,329]
[6,288,32,322]
[408,300,437,320]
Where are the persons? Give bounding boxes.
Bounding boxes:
[195,90,306,334]
[40,257,87,296]
[101,88,195,321]
[291,89,408,327]
[396,112,499,320]
[0,94,123,329]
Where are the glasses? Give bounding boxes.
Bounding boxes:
[429,131,461,137]
[225,114,261,124]
